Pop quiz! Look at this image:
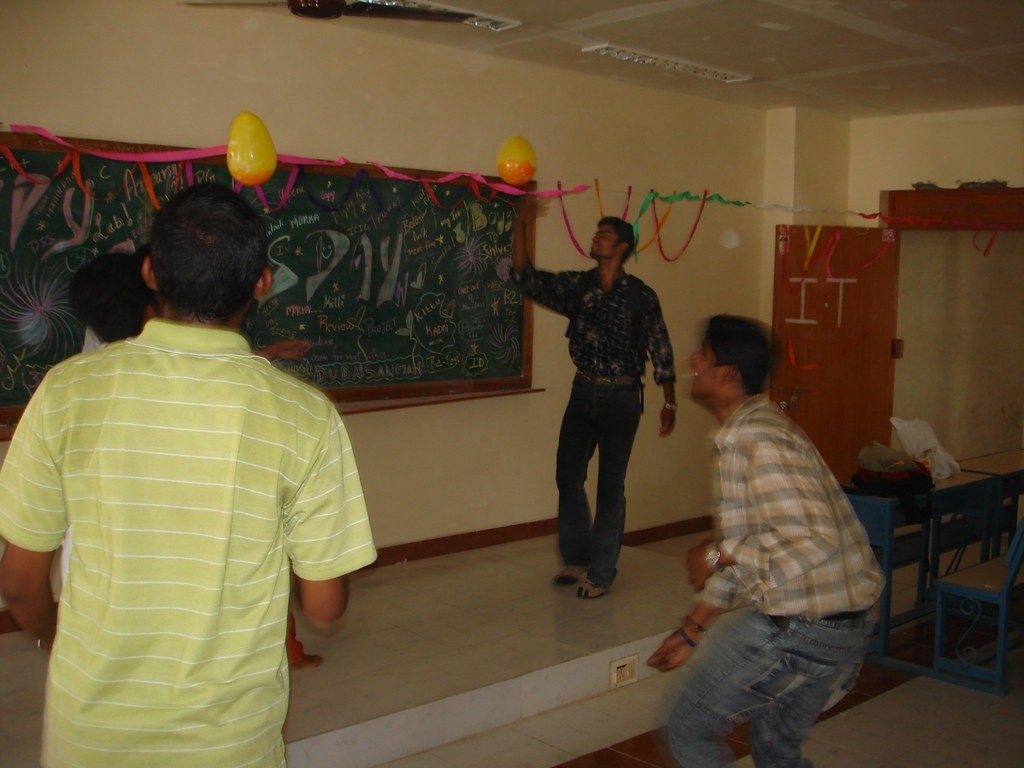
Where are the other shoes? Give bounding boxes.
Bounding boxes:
[577,579,604,600]
[556,565,585,584]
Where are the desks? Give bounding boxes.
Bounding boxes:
[835,448,1024,667]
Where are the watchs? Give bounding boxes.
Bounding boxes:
[707,536,725,572]
[666,403,678,411]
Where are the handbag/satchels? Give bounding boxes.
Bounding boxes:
[889,415,959,481]
[852,459,937,524]
[857,441,917,472]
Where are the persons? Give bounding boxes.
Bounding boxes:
[508,204,679,599]
[0,181,378,768]
[646,313,888,768]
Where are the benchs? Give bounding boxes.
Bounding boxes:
[901,514,1024,697]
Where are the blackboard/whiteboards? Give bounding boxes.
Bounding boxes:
[0,130,546,444]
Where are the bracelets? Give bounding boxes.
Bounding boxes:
[677,617,707,648]
[35,638,48,658]
